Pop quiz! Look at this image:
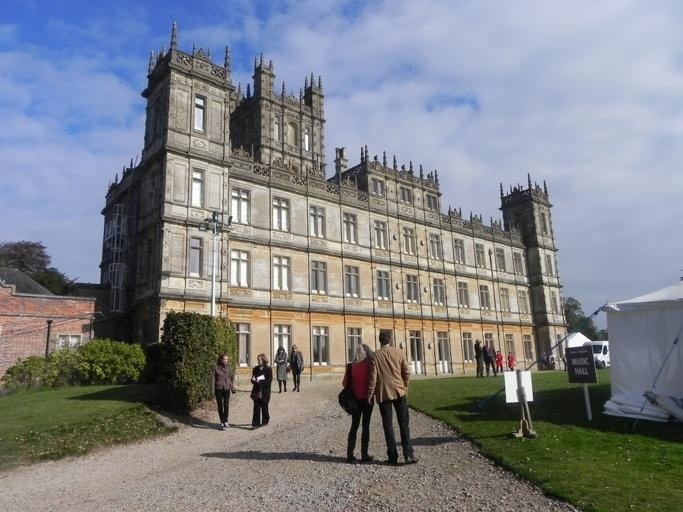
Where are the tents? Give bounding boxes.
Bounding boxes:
[565,331,592,348]
[598,275,683,423]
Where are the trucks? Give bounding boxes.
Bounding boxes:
[582,341,611,369]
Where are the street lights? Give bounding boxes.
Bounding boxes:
[196,208,232,316]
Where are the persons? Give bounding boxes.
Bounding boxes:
[472,337,484,379]
[367,328,418,465]
[340,343,374,461]
[287,344,305,393]
[249,352,273,431]
[506,351,514,371]
[541,350,547,370]
[495,349,504,374]
[483,339,497,378]
[273,345,288,393]
[209,353,236,428]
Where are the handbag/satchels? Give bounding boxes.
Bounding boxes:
[250,389,262,400]
[338,386,359,415]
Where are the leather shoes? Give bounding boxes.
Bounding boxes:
[405,457,418,463]
[362,456,374,461]
[348,455,356,460]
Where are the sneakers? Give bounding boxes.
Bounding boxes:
[221,422,230,429]
[382,459,398,466]
[253,425,258,429]
[261,423,266,426]
[279,387,299,393]
